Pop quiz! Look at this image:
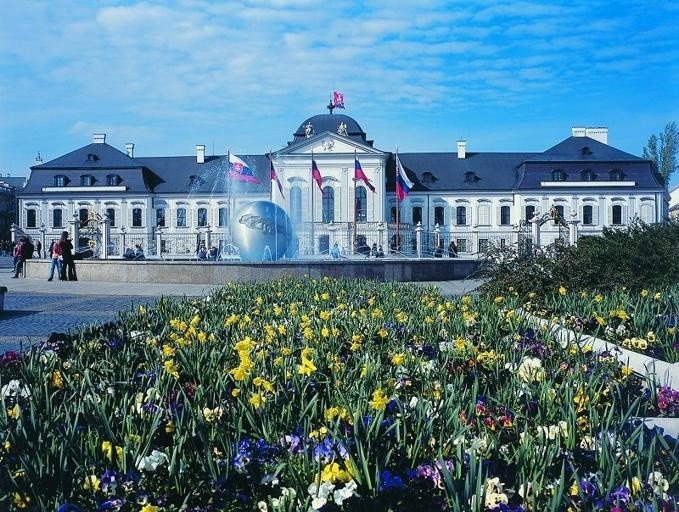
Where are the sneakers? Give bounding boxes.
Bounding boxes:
[11,269,18,278]
[48,277,77,281]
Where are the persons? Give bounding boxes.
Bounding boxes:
[434,246,443,258]
[0,231,77,282]
[448,240,458,258]
[330,241,340,258]
[302,121,314,139]
[198,244,224,261]
[335,121,348,137]
[361,241,371,256]
[134,243,146,261]
[378,245,385,256]
[372,243,378,256]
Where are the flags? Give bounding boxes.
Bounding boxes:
[395,146,415,203]
[331,89,346,109]
[310,146,326,196]
[352,149,376,193]
[227,144,262,184]
[268,147,286,201]
